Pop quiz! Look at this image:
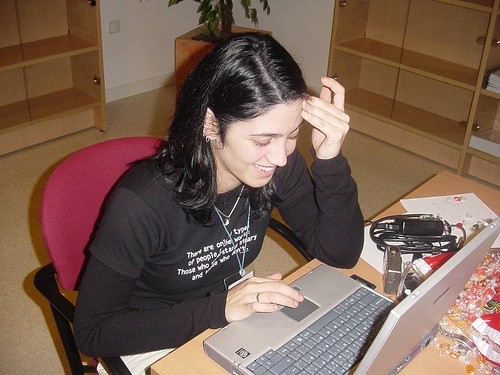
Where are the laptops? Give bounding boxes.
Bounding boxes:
[202,215,500,375]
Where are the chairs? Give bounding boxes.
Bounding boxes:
[33,137,313,375]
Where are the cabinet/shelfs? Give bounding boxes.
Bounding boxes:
[326,0,500,190]
[0,0,107,157]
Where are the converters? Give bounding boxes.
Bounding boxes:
[393,218,444,236]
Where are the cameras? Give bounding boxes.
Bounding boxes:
[384,245,401,295]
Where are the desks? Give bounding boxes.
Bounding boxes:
[149,170,500,375]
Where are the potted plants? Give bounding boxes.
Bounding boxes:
[168,0,272,101]
